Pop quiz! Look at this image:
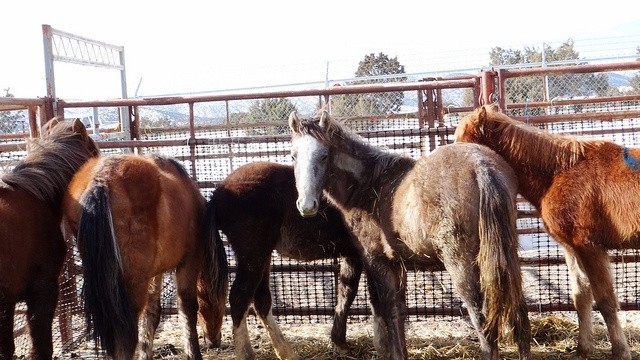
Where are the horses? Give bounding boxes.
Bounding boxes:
[208,161,364,360]
[64,151,230,360]
[288,110,531,360]
[453,100,640,360]
[0,116,101,360]
